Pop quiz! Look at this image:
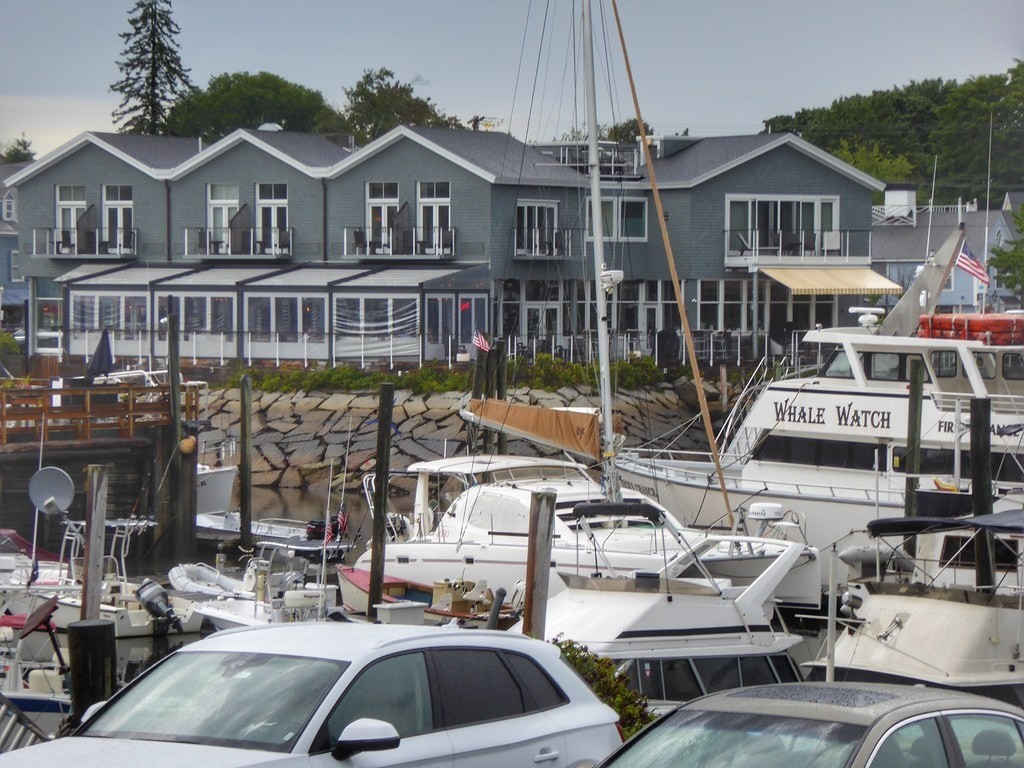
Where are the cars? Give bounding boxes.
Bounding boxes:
[590,680,1024,768]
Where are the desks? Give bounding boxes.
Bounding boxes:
[50,240,65,253]
[366,240,383,253]
[97,240,113,253]
[416,240,432,253]
[252,240,270,255]
[208,241,224,254]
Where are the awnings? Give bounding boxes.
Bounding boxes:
[759,266,903,295]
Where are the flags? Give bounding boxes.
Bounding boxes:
[337,502,349,534]
[472,328,491,353]
[955,242,990,286]
[325,512,332,544]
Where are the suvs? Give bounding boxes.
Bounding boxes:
[0,620,624,768]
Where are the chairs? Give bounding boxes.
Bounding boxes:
[971,728,1017,768]
[797,232,816,255]
[737,233,780,258]
[119,233,134,255]
[354,231,367,254]
[197,232,210,254]
[277,232,290,256]
[821,229,841,255]
[61,231,74,254]
[437,231,454,255]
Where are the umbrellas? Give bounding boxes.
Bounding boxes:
[85,327,114,383]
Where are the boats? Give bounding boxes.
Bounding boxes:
[491,497,824,717]
[166,523,528,633]
[0,522,190,640]
[7,0,1024,702]
[335,445,707,631]
[799,504,1024,705]
